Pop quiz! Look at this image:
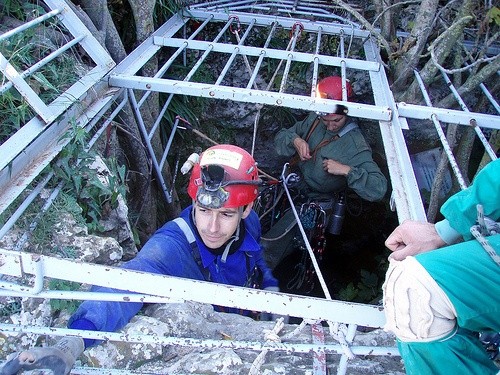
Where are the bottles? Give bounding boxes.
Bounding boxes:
[329,196,347,235]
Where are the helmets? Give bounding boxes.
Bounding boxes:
[181,144,259,209]
[314,76,356,116]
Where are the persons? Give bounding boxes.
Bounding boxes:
[262,77,387,289]
[0,144,287,375]
[383,158,499,375]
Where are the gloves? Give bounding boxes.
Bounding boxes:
[0,336,85,375]
